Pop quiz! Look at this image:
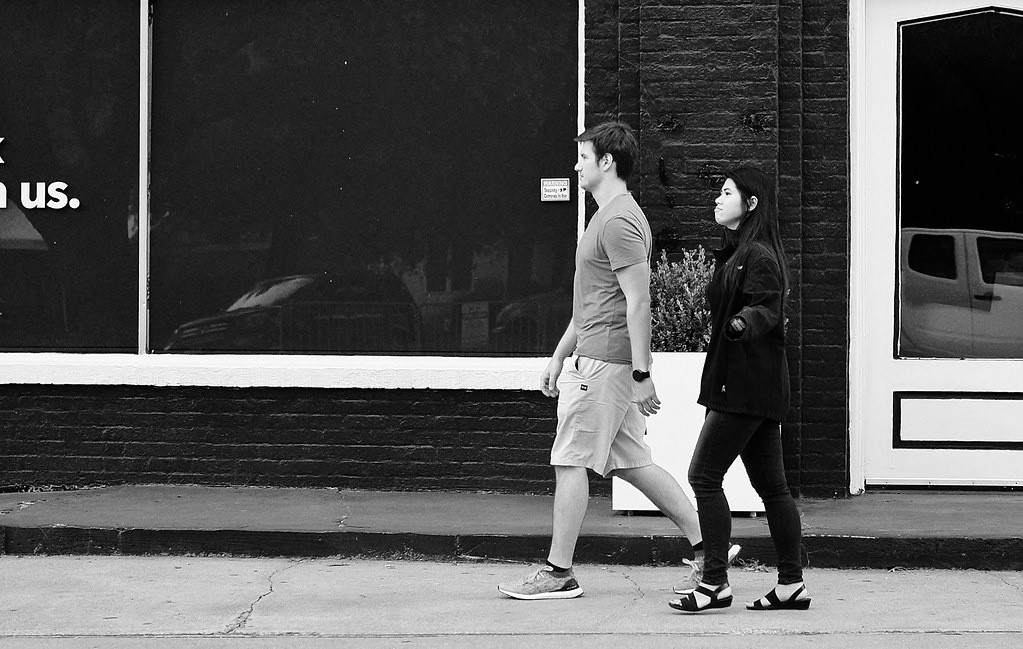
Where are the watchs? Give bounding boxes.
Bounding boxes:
[632,369,650,382]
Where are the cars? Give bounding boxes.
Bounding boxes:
[901,227,1022,358]
[491,254,575,352]
[167,272,423,355]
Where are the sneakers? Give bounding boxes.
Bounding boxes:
[672,541,744,594]
[497,563,585,600]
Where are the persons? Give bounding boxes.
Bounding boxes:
[497,120,741,600]
[667,166,812,612]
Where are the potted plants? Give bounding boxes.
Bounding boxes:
[610,244,768,516]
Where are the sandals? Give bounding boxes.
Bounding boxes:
[668,582,733,612]
[746,584,811,610]
